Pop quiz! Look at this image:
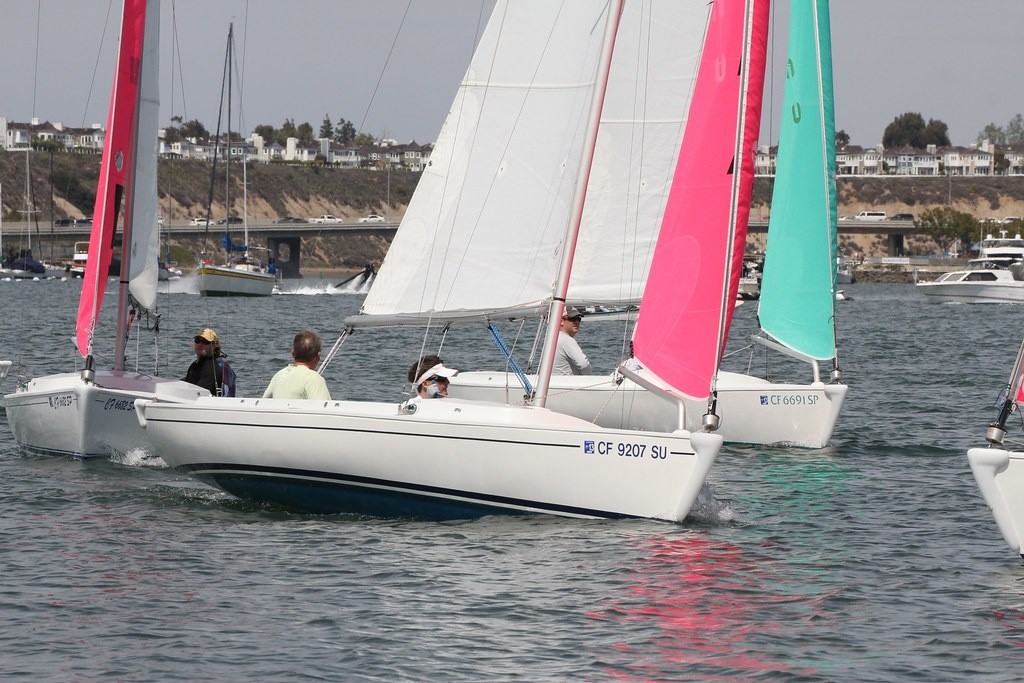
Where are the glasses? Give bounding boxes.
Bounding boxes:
[563,317,581,323]
[195,339,210,345]
[426,376,447,382]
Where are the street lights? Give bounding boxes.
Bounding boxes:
[377,159,390,222]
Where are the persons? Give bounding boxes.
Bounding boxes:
[550,306,592,375]
[263,330,331,400]
[404,355,459,404]
[365,264,374,281]
[181,328,236,397]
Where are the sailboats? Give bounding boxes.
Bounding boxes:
[195,23,276,298]
[134,0,769,525]
[0,147,67,279]
[0,0,172,460]
[966,339,1024,562]
[445,0,849,448]
[158,181,183,281]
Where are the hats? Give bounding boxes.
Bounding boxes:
[415,364,459,385]
[215,340,220,348]
[193,328,216,345]
[562,305,584,318]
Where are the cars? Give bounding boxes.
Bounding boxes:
[55,220,92,227]
[855,212,914,222]
[190,218,243,226]
[357,215,385,223]
[979,217,1020,224]
[273,217,307,223]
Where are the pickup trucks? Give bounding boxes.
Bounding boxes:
[308,215,343,224]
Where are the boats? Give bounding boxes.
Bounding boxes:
[69,242,90,279]
[736,279,761,301]
[916,230,1024,304]
[575,305,640,322]
[836,255,855,284]
